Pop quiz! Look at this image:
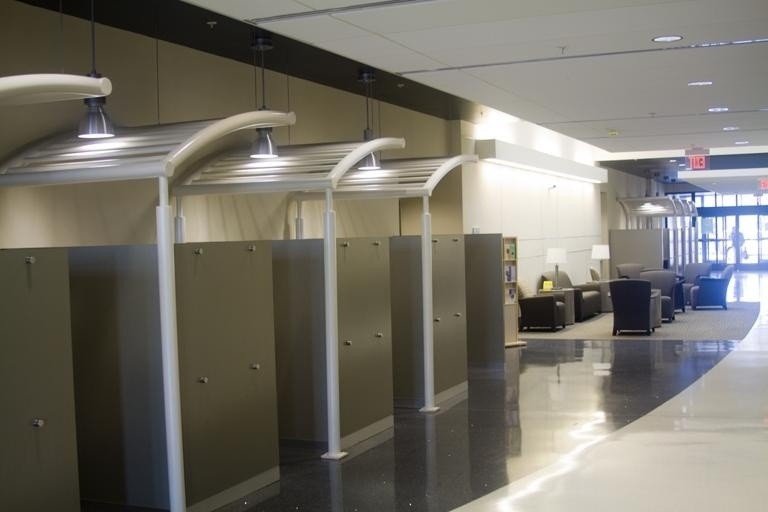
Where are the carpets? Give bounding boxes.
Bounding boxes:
[518,301,760,341]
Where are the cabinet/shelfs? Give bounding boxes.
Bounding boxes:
[503,236,526,347]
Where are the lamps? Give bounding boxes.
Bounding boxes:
[76,1,116,139]
[356,71,382,172]
[249,38,280,159]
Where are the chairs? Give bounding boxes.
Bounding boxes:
[518,262,734,336]
